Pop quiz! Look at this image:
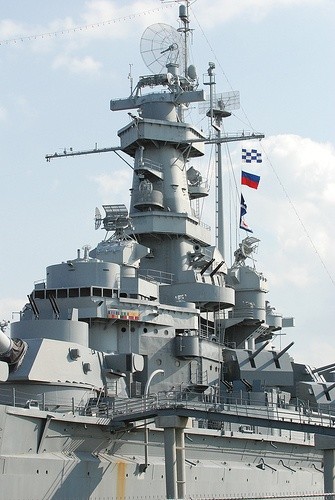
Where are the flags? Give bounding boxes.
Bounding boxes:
[240,218,252,232]
[242,170,261,190]
[239,192,247,225]
[241,147,263,164]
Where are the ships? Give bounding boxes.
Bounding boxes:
[1,0,334,500]
